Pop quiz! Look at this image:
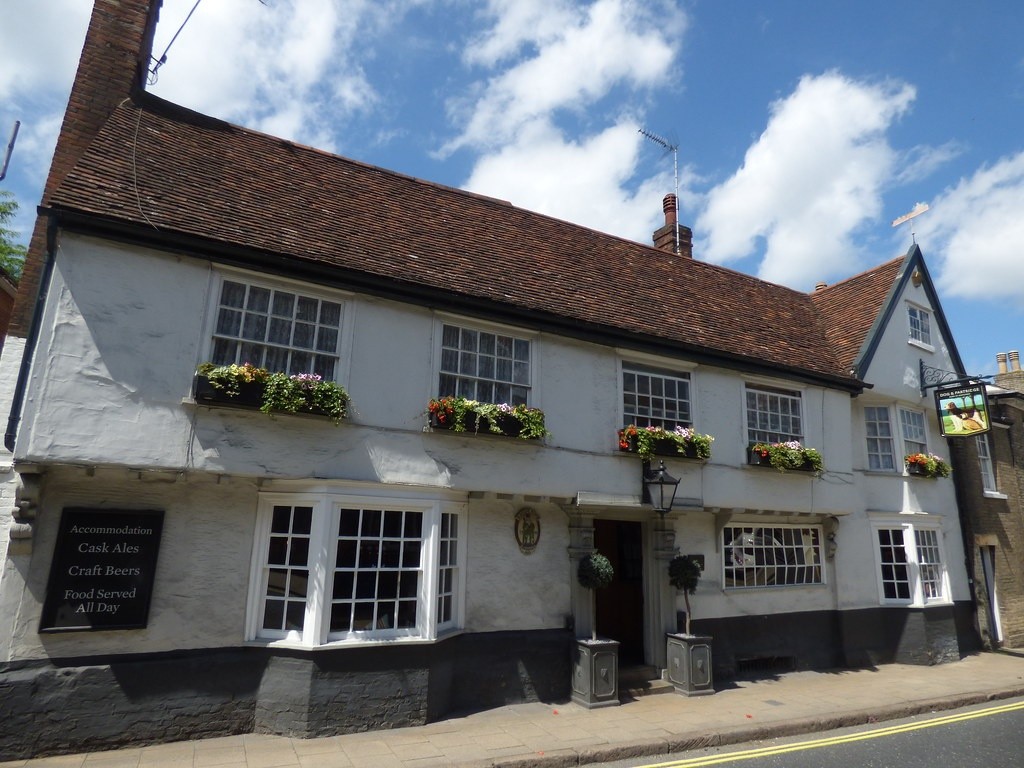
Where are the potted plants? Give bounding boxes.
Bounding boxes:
[570,553,621,708]
[667,554,715,694]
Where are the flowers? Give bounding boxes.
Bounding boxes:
[616,425,713,460]
[261,373,351,427]
[752,440,823,477]
[905,453,953,477]
[192,361,270,397]
[418,398,554,441]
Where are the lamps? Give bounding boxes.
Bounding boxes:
[643,459,682,534]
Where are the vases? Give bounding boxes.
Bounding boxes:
[192,374,258,406]
[906,462,942,477]
[750,452,812,470]
[299,399,331,416]
[431,411,524,436]
[628,438,699,458]
